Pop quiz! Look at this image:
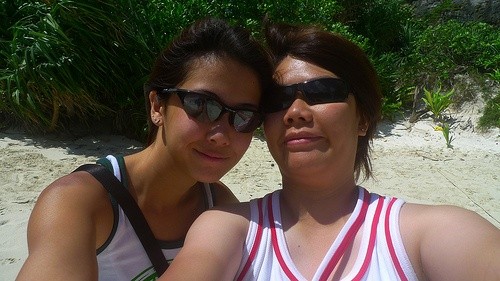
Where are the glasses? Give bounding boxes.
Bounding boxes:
[261,77,354,112]
[158,88,263,134]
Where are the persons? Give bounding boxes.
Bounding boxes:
[154,21,500,281]
[14,22,278,281]
